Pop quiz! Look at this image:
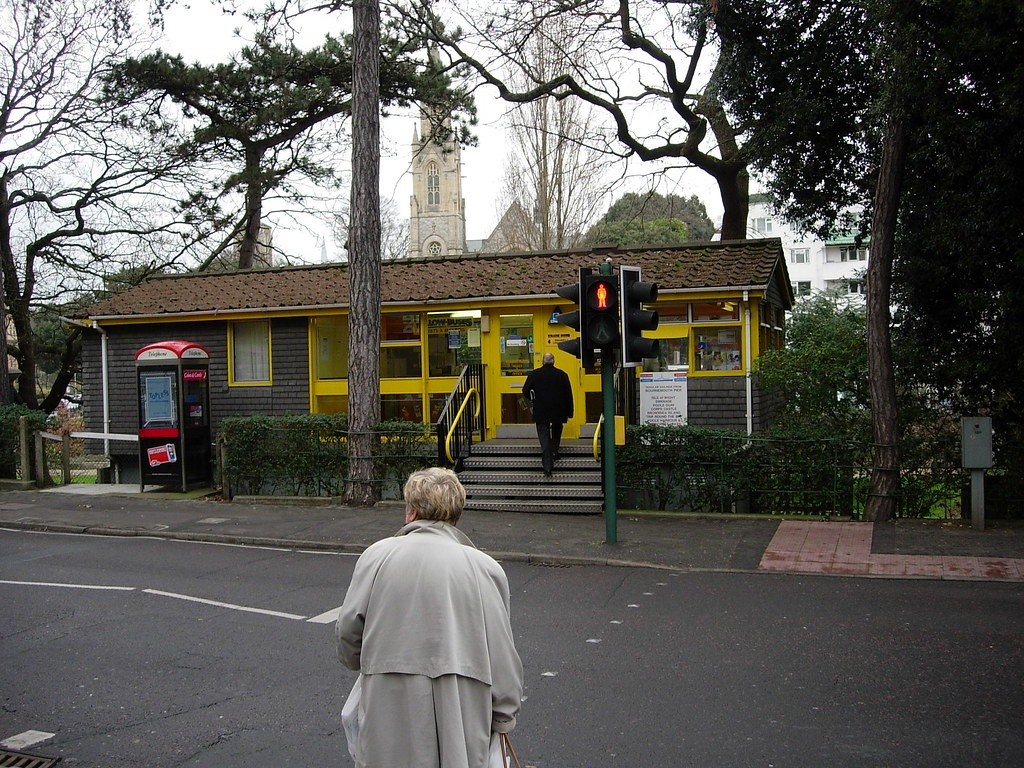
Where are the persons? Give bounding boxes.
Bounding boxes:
[335,467,524,768]
[522,353,574,476]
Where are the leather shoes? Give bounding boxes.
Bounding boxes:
[544,451,560,479]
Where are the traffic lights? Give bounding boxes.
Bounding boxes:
[619,265,659,369]
[553,267,597,370]
[586,273,620,351]
